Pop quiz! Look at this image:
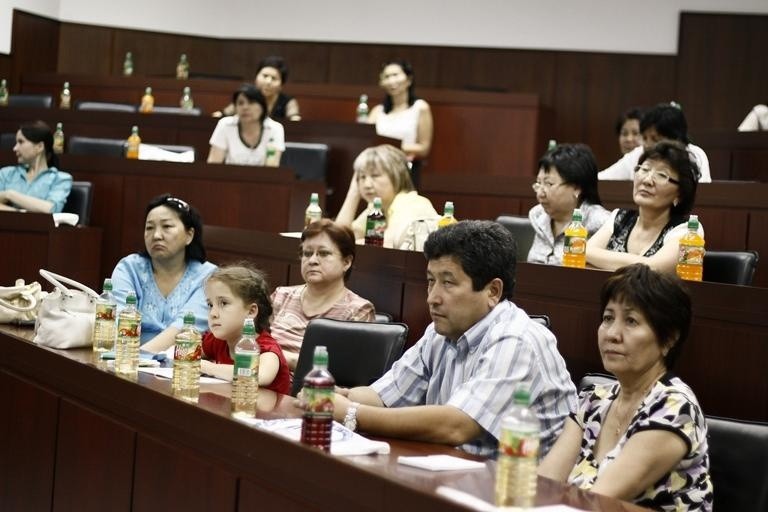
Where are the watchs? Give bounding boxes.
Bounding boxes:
[342,401,361,433]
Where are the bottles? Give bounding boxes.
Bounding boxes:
[304,191,459,247]
[264,137,277,166]
[296,343,337,450]
[673,213,705,281]
[60,81,72,109]
[1,79,10,107]
[111,288,143,378]
[357,94,369,117]
[562,210,586,271]
[180,86,194,109]
[94,275,118,356]
[126,126,142,158]
[122,51,134,76]
[139,86,154,114]
[176,53,190,80]
[493,390,540,501]
[170,308,204,407]
[228,314,261,420]
[51,122,65,153]
[543,139,560,154]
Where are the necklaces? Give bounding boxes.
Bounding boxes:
[614,385,647,436]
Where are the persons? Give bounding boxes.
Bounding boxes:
[537,262,715,512]
[0,119,74,212]
[207,84,286,168]
[334,143,441,252]
[364,57,434,190]
[527,101,711,279]
[110,192,220,355]
[266,221,376,386]
[198,261,290,394]
[210,55,302,121]
[293,219,579,472]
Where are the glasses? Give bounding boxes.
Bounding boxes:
[531,180,568,194]
[634,163,682,187]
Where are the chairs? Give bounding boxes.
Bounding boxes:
[77,101,137,113]
[62,182,93,225]
[528,313,551,330]
[66,136,196,164]
[495,215,535,262]
[703,250,760,285]
[289,312,409,389]
[152,106,205,117]
[7,95,54,109]
[281,142,329,181]
[705,414,767,509]
[578,372,619,393]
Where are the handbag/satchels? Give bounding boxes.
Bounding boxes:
[33,269,98,349]
[0,278,49,324]
[398,218,441,252]
